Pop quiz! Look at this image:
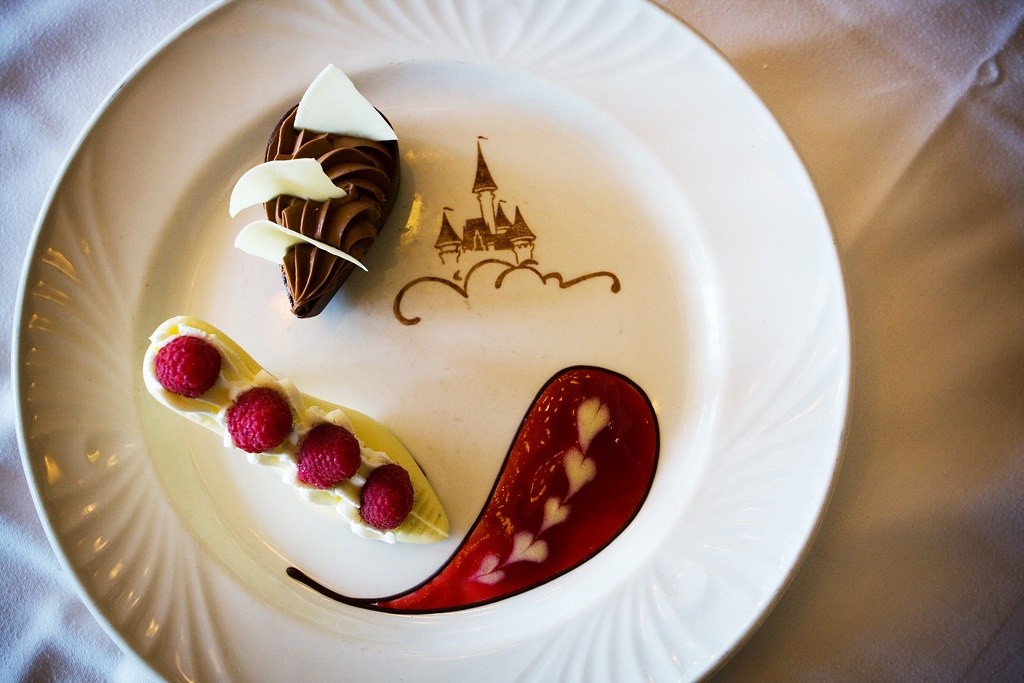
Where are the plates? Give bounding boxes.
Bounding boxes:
[11,1,851,682]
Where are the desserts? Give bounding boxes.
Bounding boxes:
[144,318,453,546]
[228,63,402,319]
